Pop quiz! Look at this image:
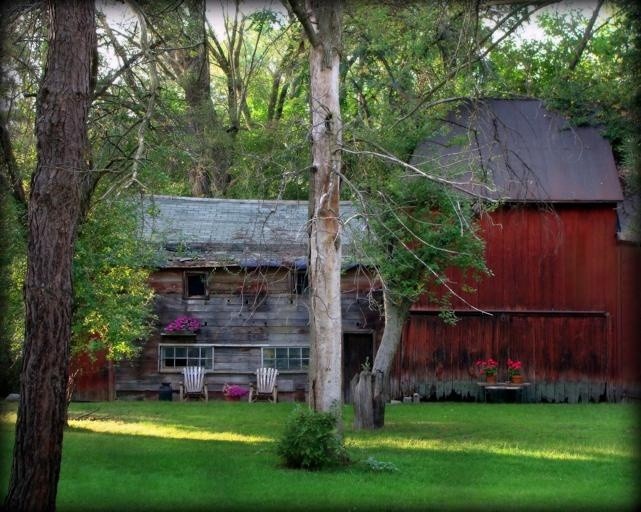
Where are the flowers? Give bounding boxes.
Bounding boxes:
[223,383,248,398]
[162,315,201,333]
[475,357,500,376]
[504,357,523,376]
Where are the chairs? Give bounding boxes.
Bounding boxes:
[178,365,209,402]
[247,366,280,404]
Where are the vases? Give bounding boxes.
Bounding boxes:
[485,376,497,384]
[224,395,240,401]
[511,375,523,384]
